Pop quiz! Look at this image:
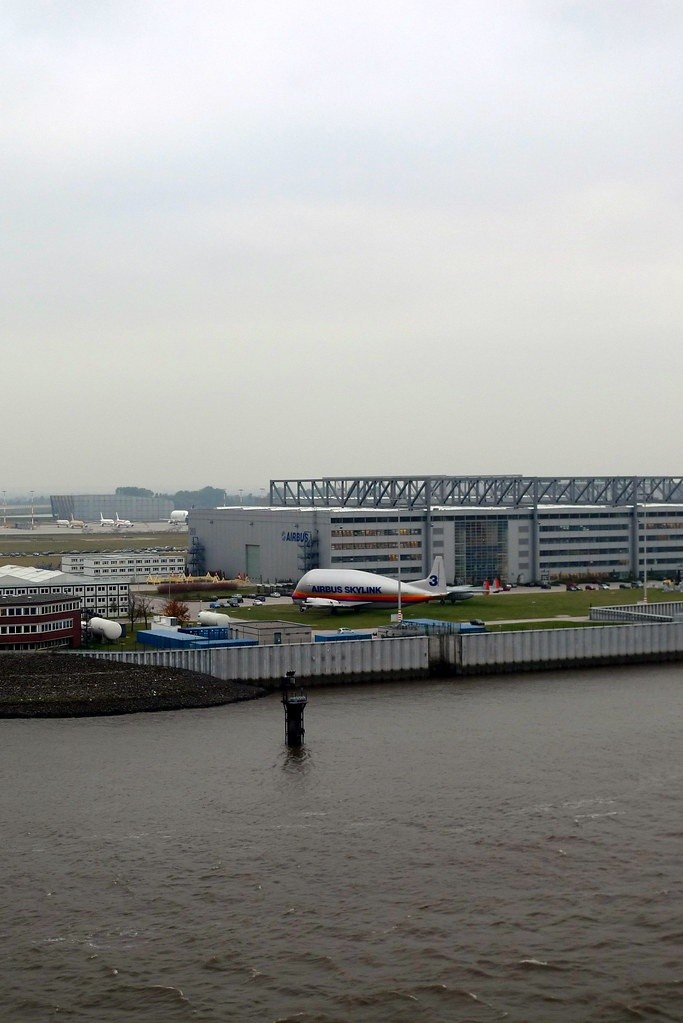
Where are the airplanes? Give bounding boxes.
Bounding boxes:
[113,510,135,529]
[160,509,190,524]
[56,520,70,529]
[289,556,504,617]
[68,512,87,530]
[98,512,116,529]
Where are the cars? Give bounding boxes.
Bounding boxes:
[269,592,281,598]
[255,594,267,601]
[219,602,231,608]
[209,603,220,608]
[227,593,244,607]
[566,586,582,592]
[620,583,631,589]
[599,584,610,590]
[585,585,596,590]
[253,600,263,606]
[631,581,644,588]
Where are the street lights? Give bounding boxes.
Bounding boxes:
[238,489,244,505]
[2,490,7,523]
[29,490,35,526]
